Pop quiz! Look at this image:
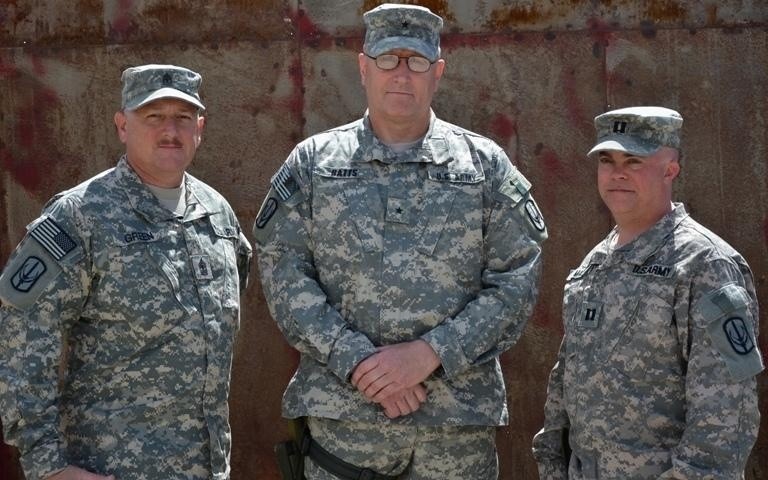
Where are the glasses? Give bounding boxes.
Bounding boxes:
[364,54,439,73]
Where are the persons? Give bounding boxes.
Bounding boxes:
[1,62,256,480]
[249,2,555,479]
[527,102,767,480]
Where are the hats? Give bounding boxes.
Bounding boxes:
[120,62,206,112]
[362,4,444,62]
[586,105,684,158]
